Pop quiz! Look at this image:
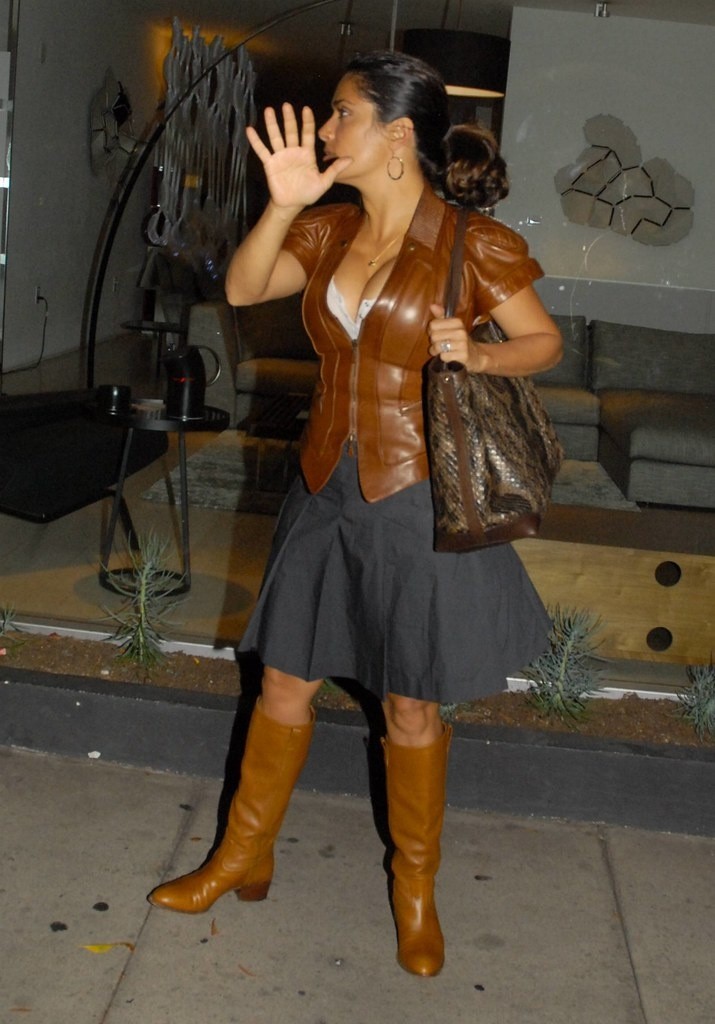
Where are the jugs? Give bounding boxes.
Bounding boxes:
[157,344,222,420]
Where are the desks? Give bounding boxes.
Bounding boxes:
[93,400,230,598]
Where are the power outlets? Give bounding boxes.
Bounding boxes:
[34,286,41,304]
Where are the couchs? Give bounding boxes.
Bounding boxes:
[185,293,715,510]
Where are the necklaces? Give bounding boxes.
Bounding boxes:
[366,214,410,266]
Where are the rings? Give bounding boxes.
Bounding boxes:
[441,341,451,352]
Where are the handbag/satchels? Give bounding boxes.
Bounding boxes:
[424,207,564,552]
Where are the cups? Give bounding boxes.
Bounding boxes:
[98,383,133,416]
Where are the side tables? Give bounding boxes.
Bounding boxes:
[121,320,182,387]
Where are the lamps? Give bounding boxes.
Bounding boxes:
[404,0,511,99]
[595,0,611,17]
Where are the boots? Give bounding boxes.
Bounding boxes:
[381,719,452,978]
[147,695,316,915]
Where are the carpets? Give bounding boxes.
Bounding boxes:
[137,429,643,515]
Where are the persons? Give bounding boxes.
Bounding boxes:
[148,53,563,977]
